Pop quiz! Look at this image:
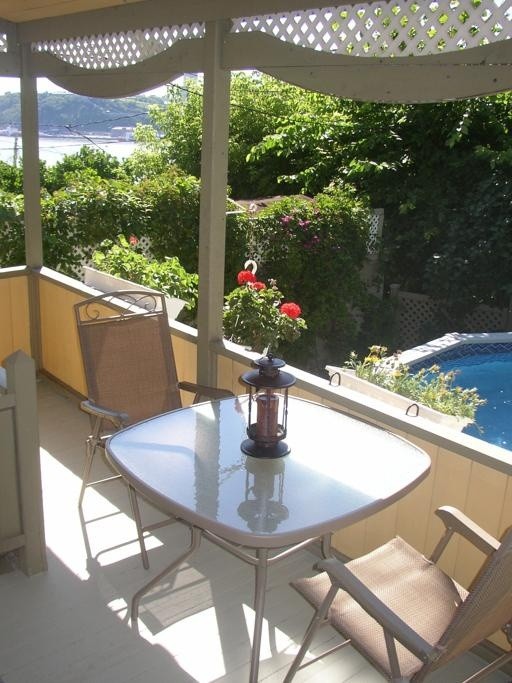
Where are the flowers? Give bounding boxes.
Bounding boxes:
[342,343,488,436]
[222,271,307,354]
[87,234,198,314]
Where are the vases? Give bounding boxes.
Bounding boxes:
[82,265,187,321]
[325,363,474,432]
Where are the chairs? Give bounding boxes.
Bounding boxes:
[285,506,511,682]
[73,291,238,570]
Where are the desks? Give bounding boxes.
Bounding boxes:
[105,391,433,683]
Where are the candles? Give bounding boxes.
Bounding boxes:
[257,394,279,441]
